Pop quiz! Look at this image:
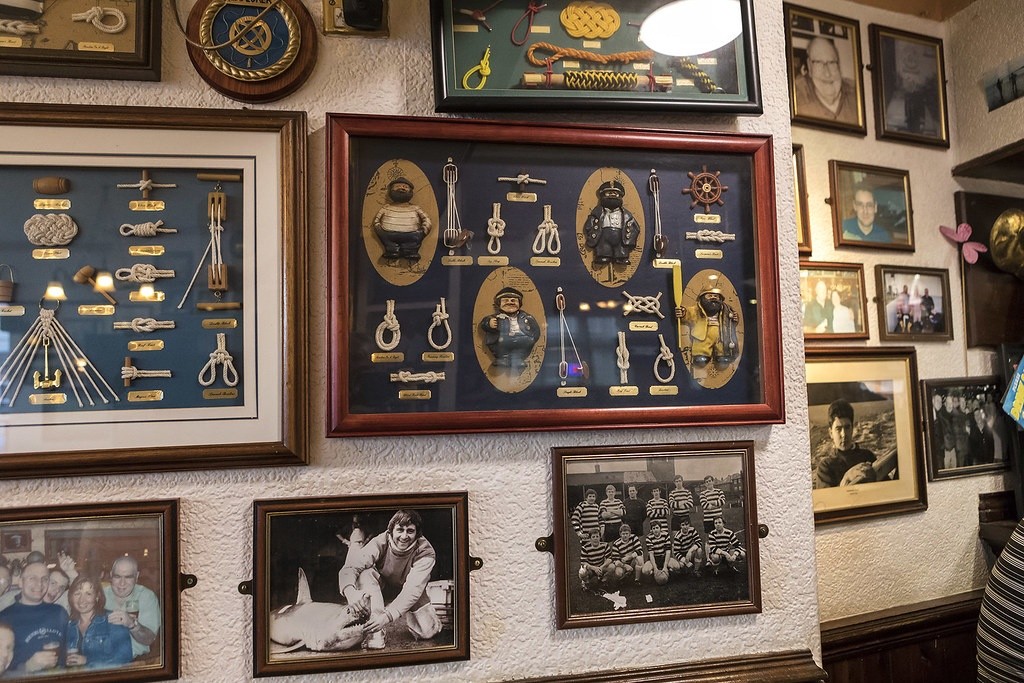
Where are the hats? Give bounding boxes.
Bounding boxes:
[620,524,631,532]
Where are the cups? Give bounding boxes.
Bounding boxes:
[43,641,60,656]
[65,647,79,666]
[126,600,139,621]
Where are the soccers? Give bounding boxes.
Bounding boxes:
[655,571,669,585]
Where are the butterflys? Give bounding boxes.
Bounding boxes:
[938,222,988,264]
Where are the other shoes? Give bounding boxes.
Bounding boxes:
[365,626,390,649]
[645,594,653,603]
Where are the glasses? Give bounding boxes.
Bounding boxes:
[705,479,711,483]
[855,201,874,211]
[810,60,838,72]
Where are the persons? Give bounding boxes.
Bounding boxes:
[887,78,925,134]
[571,475,746,592]
[932,385,1002,470]
[815,399,891,489]
[996,78,1003,99]
[1,551,161,679]
[338,509,443,649]
[795,37,857,123]
[842,186,891,243]
[802,282,855,333]
[897,285,938,334]
[1008,73,1017,93]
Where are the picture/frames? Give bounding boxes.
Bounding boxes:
[0,0,164,87]
[874,261,958,344]
[783,0,873,140]
[246,486,472,680]
[0,103,309,487]
[825,157,919,256]
[797,258,870,342]
[800,344,934,530]
[0,496,185,683]
[918,374,1016,485]
[546,436,766,639]
[787,142,816,256]
[320,109,796,442]
[426,0,774,117]
[867,16,953,150]
